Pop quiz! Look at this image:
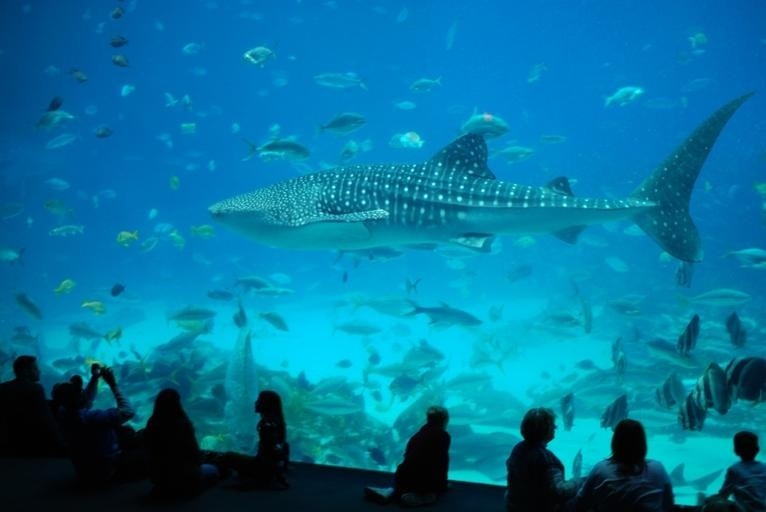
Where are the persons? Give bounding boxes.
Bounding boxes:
[1,356,202,510]
[682,432,766,512]
[392,408,450,506]
[559,420,674,512]
[504,412,587,511]
[206,392,289,493]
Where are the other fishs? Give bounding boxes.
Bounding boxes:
[0,1,766,507]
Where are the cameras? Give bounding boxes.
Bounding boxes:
[93,365,110,376]
